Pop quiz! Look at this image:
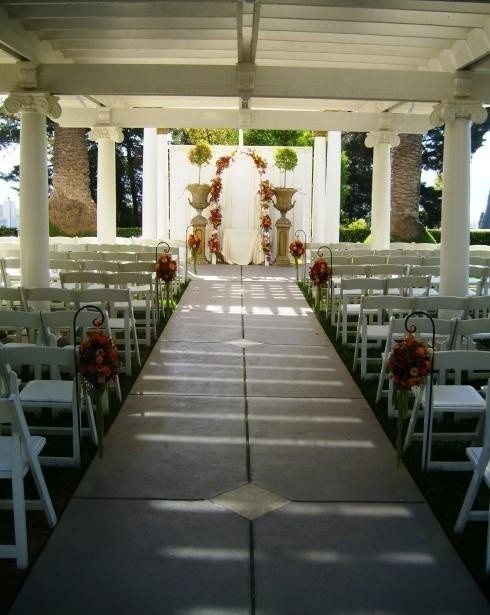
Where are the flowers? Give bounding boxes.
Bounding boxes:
[209,147,275,267]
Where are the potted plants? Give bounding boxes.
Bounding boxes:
[185,143,211,209]
[271,148,299,212]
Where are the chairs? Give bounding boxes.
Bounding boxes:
[1,342,99,469]
[0,235,180,417]
[0,395,58,570]
[402,348,489,472]
[303,242,490,325]
[352,294,490,418]
[453,445,490,572]
